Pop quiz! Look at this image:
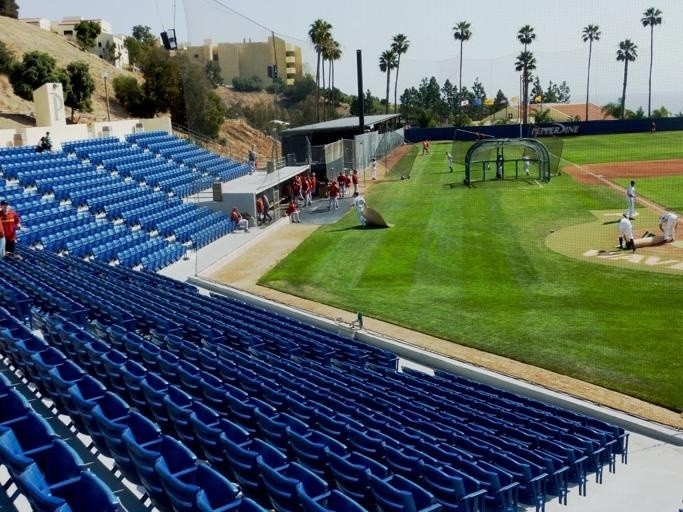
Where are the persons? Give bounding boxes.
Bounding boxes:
[651,121,656,133]
[496,155,505,178]
[0,200,21,259]
[354,192,368,226]
[522,153,530,175]
[618,217,636,252]
[445,152,454,173]
[623,180,637,219]
[282,170,359,223]
[36,131,53,152]
[231,207,251,233]
[256,193,272,223]
[248,149,256,171]
[658,212,680,243]
[370,158,377,180]
[423,139,431,155]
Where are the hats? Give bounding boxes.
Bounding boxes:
[1,200,10,206]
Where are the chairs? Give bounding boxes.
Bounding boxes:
[1,245,632,512]
[0,129,254,274]
